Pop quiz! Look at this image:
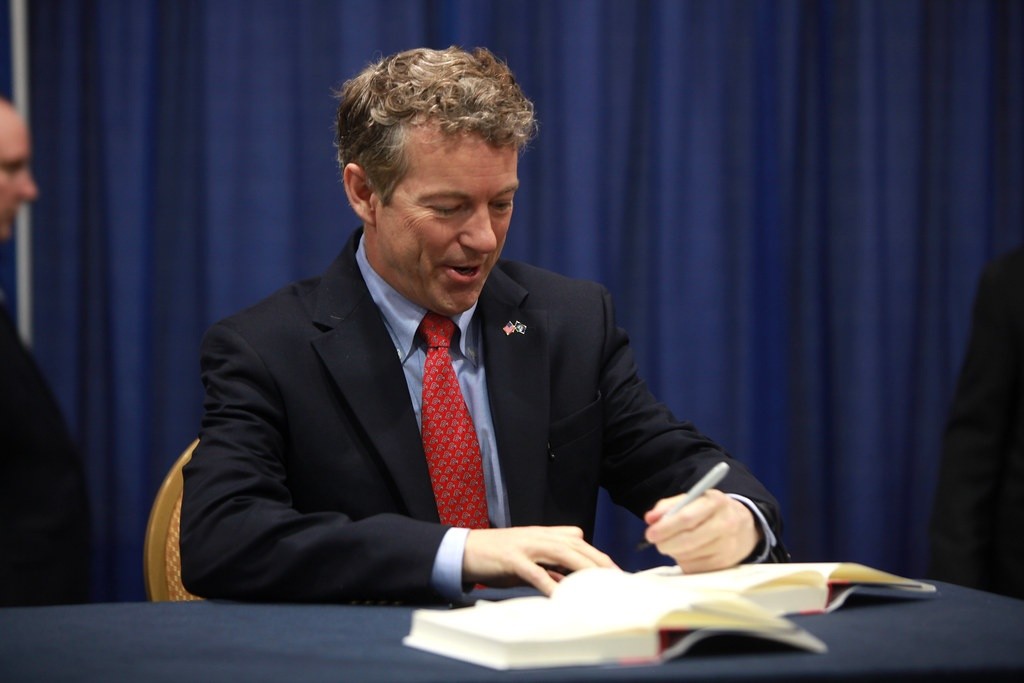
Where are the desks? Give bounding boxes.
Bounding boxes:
[2,577,1022,683]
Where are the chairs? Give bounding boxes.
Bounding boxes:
[144,437,210,602]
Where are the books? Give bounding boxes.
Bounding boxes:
[637,561,936,615]
[403,567,828,670]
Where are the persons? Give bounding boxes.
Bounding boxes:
[0,101,95,608]
[179,45,786,606]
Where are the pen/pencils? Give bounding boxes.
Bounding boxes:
[633,455,740,555]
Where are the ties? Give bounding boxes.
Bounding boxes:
[416,314,487,534]
